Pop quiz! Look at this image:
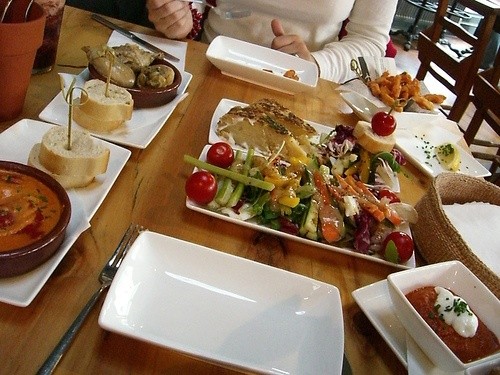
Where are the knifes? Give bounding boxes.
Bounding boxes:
[91,14,180,63]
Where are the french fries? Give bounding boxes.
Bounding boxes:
[371,72,446,113]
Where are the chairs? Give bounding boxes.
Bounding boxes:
[463,41,500,184]
[416,1,500,121]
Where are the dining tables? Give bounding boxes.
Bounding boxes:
[0,0,500,375]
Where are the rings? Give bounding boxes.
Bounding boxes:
[295,53,299,57]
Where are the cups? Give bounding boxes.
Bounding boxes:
[0,0,46,122]
[32,0,65,75]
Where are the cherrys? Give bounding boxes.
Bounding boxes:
[372,111,397,136]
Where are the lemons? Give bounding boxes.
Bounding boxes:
[435,143,460,171]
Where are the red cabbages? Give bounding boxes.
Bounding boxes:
[324,124,407,254]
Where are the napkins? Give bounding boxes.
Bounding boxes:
[109,27,188,70]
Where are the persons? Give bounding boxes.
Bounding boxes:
[146,0,399,85]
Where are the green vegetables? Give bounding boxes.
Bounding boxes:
[251,152,400,261]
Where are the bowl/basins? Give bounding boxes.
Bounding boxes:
[0,160,71,279]
[387,260,500,374]
[360,88,440,130]
[89,59,181,108]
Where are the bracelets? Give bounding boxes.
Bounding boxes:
[187,6,202,40]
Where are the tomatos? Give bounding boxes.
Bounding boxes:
[185,171,218,204]
[206,142,234,168]
[383,231,414,262]
[380,189,401,204]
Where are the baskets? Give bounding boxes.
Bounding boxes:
[410,171,500,299]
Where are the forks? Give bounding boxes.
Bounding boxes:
[35,225,149,375]
[183,0,252,19]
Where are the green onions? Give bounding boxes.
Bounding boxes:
[415,134,469,171]
[429,298,472,320]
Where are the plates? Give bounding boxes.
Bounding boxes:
[352,260,500,375]
[207,98,401,194]
[38,56,193,149]
[97,231,344,375]
[0,120,131,308]
[339,83,491,178]
[206,35,318,95]
[185,143,417,268]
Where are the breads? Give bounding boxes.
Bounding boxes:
[353,121,396,155]
[73,79,133,134]
[213,98,320,160]
[28,127,109,190]
